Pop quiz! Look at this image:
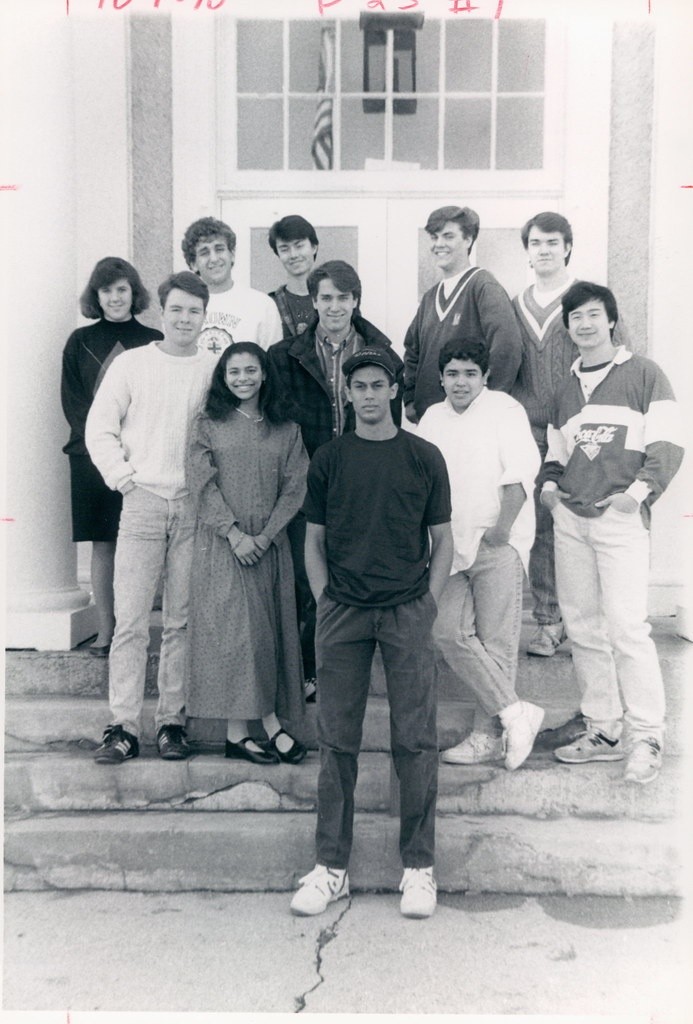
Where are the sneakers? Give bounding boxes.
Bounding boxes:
[399,866,437,919]
[551,726,624,763]
[93,723,139,763]
[290,863,349,916]
[158,723,189,758]
[624,736,662,783]
[441,731,504,764]
[499,700,545,770]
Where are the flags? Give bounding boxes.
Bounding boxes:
[310,27,332,171]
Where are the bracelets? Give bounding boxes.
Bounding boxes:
[230,531,243,553]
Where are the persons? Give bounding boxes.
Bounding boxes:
[62,205,683,783]
[288,345,453,917]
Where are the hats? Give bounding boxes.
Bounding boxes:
[341,346,395,381]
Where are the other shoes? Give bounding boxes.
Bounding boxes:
[224,727,306,765]
[526,622,567,657]
[89,638,110,657]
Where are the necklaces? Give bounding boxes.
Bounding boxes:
[231,405,264,423]
